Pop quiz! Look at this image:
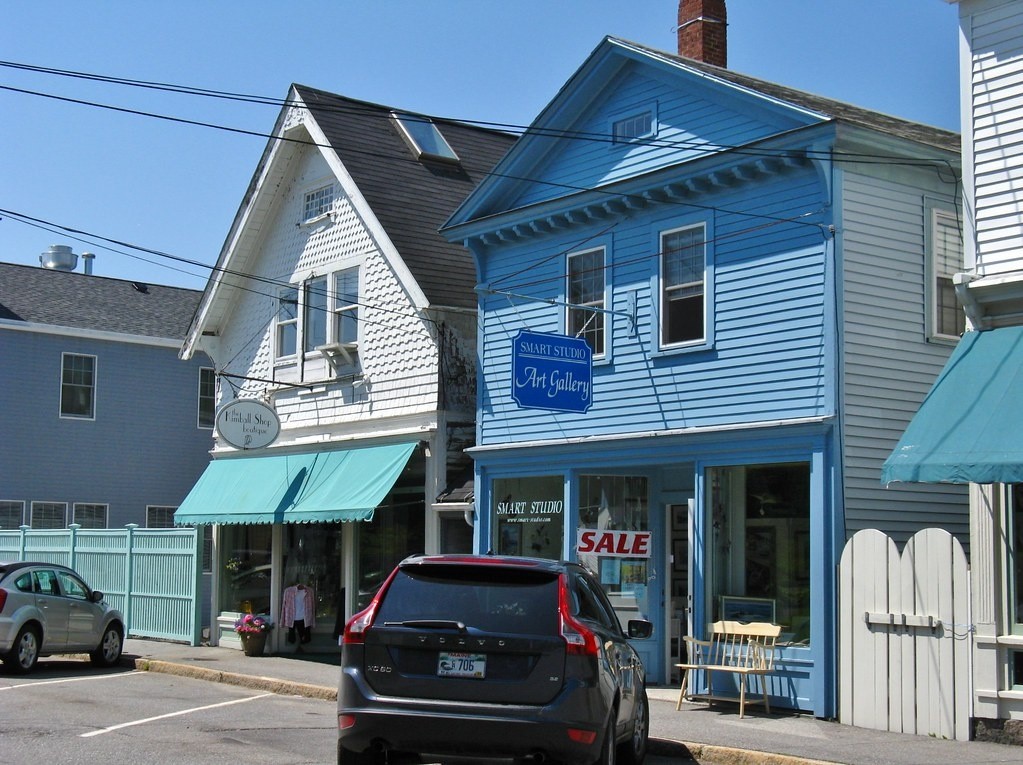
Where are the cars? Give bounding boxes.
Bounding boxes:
[0,561,126,676]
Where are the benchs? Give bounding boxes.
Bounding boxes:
[672,620,784,718]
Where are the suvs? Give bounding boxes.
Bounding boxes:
[336,551,650,764]
